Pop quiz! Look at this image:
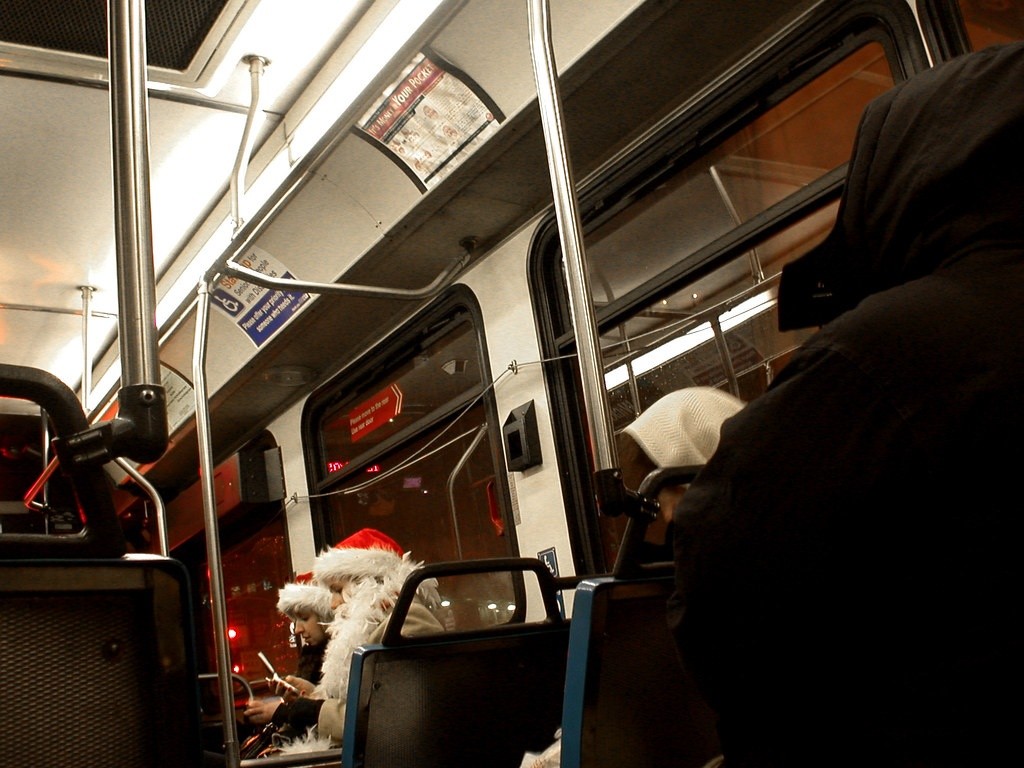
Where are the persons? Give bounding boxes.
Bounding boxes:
[232,42,1024,765]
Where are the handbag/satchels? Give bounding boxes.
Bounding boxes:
[240,722,276,760]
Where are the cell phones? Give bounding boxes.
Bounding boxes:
[257,652,300,698]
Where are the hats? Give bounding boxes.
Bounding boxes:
[311,528,441,609]
[277,571,333,628]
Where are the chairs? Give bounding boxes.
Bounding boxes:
[1,363,708,768]
